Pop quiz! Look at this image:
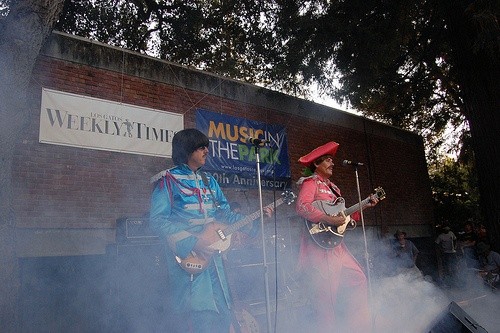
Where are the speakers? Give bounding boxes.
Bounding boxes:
[426,301,488,333]
[102,244,167,325]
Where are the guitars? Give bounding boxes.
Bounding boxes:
[304,186,386,250]
[173,190,298,274]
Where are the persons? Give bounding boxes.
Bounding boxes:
[295,140,369,333]
[229,201,243,248]
[387,223,500,333]
[150,128,275,333]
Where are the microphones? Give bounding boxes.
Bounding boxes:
[343,160,364,166]
[245,136,270,144]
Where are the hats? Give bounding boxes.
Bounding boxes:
[299,140,339,167]
[394,229,406,239]
[172,128,209,159]
